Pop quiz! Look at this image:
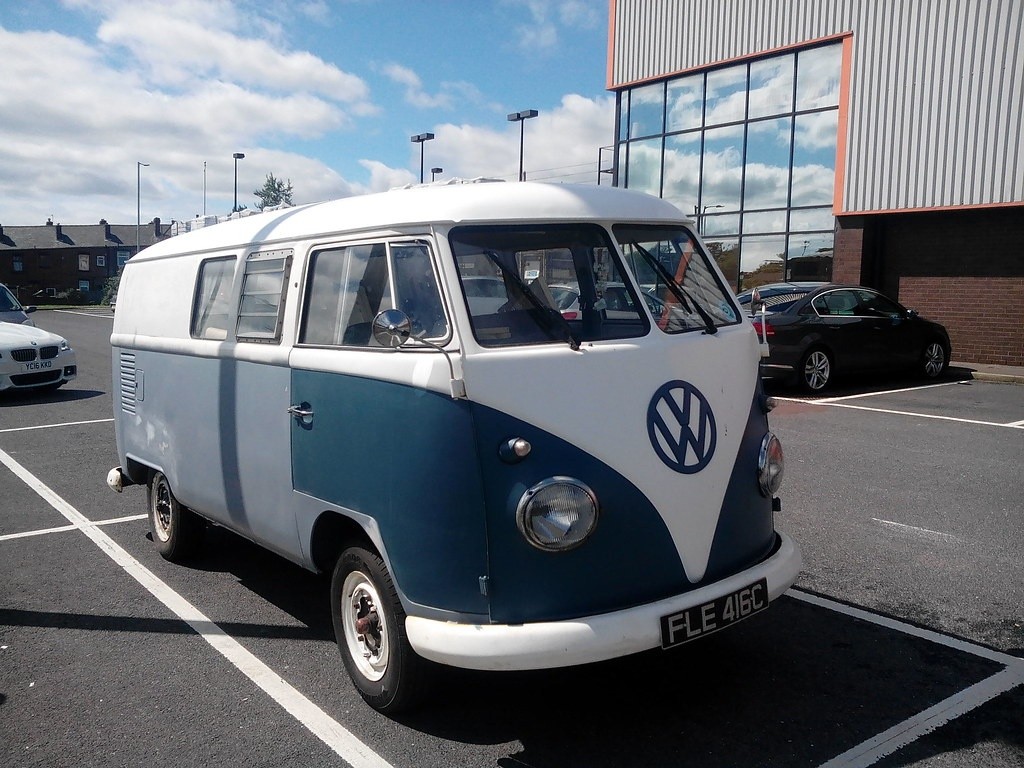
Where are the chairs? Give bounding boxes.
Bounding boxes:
[828,296,845,315]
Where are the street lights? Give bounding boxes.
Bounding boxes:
[137,162,150,253]
[431,168,443,182]
[411,133,434,184]
[232,153,245,211]
[507,109,538,181]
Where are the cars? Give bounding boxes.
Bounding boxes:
[0,321,77,395]
[720,282,900,321]
[751,284,951,395]
[0,284,37,327]
[462,276,508,315]
[107,182,801,712]
[548,281,696,319]
